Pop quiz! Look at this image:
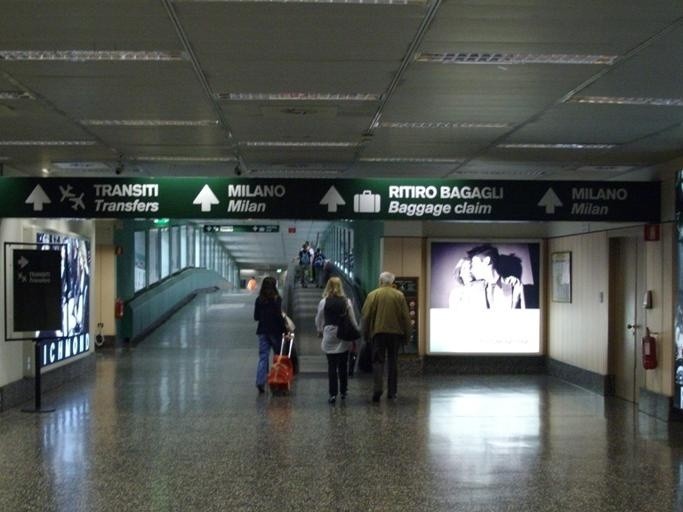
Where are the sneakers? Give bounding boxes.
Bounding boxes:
[340,392,347,399]
[388,393,397,399]
[256,384,264,392]
[328,396,336,403]
[373,391,382,402]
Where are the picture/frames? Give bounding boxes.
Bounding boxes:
[551,251,572,304]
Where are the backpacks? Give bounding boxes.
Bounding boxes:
[301,252,309,264]
[314,256,323,266]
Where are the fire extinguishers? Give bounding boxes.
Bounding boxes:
[115,296,124,319]
[640,327,657,369]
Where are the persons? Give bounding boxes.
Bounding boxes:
[250,277,289,394]
[306,241,314,282]
[298,243,311,287]
[316,276,359,403]
[314,248,326,289]
[362,271,412,401]
[448,247,526,308]
[250,276,257,291]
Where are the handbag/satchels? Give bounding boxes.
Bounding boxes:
[336,315,360,341]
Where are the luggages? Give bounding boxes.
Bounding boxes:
[268,333,295,392]
